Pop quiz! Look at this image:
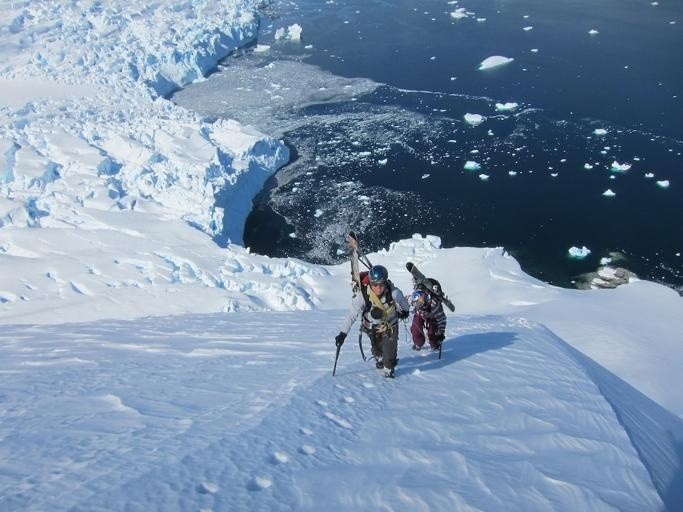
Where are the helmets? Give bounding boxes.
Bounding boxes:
[367,265,387,284]
[410,290,432,312]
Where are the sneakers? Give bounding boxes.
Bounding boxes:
[384,368,394,377]
[415,345,421,351]
[375,356,383,369]
[428,346,436,352]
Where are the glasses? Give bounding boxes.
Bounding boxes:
[371,282,386,287]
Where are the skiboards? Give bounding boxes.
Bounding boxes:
[406,262,456,312]
[347,230,361,299]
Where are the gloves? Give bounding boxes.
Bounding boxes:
[433,334,444,342]
[402,310,408,319]
[334,332,347,348]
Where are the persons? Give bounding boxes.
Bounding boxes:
[335,265,409,378]
[410,291,446,350]
